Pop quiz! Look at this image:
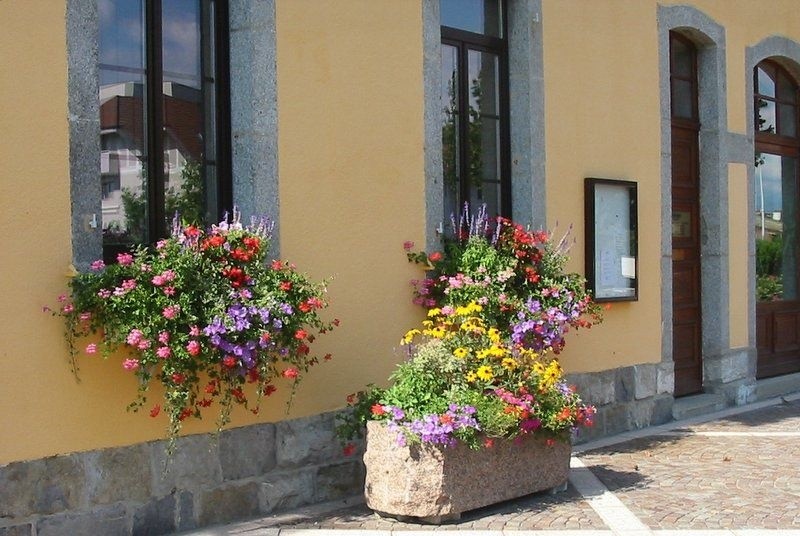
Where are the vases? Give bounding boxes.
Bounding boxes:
[364,420,572,524]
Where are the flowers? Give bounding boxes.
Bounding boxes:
[42,202,340,482]
[333,200,611,455]
